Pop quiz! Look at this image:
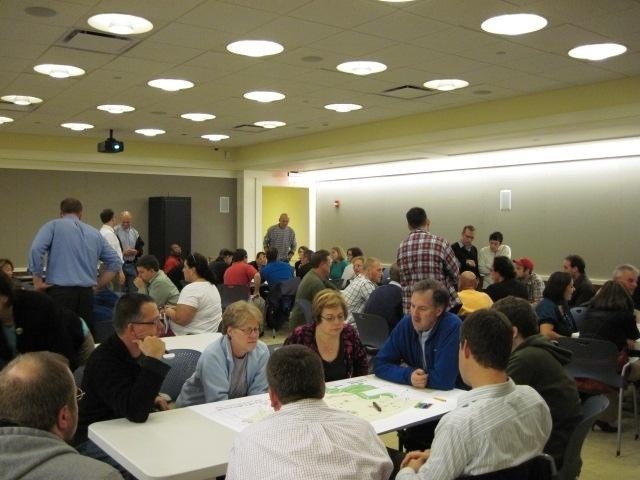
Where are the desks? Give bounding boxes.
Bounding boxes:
[88,369,482,479]
[93,333,225,365]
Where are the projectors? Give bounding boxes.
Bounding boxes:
[98,140,124,152]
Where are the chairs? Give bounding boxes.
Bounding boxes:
[454,454,557,479]
[154,348,208,395]
[350,308,396,369]
[559,336,638,459]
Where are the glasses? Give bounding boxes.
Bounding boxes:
[66,388,86,406]
[130,316,162,329]
[319,314,345,323]
[235,327,262,336]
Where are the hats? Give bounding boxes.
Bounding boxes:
[513,258,534,270]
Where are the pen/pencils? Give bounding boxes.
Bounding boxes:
[373,402,381,412]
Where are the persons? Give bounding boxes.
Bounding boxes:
[371,278,472,452]
[225,345,395,480]
[75,293,172,480]
[133,213,297,337]
[490,296,582,474]
[288,207,544,357]
[1,197,146,376]
[175,300,270,408]
[535,253,640,353]
[385,307,553,479]
[282,289,369,383]
[0,350,125,480]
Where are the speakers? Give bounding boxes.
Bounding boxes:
[220,196,229,213]
[500,190,511,211]
